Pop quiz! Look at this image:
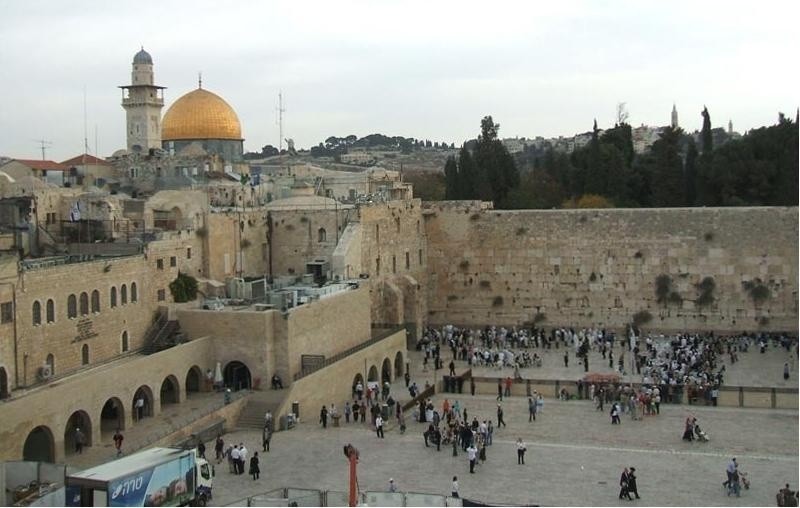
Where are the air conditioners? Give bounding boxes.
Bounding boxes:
[40,364,52,379]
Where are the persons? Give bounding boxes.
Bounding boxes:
[722,457,737,488]
[272,373,284,391]
[785,492,798,507]
[73,427,86,456]
[726,463,748,499]
[320,318,798,474]
[629,468,642,500]
[262,426,271,452]
[205,369,214,392]
[619,467,632,501]
[263,410,273,434]
[133,398,146,421]
[388,477,398,492]
[248,452,261,481]
[112,428,124,457]
[776,489,785,507]
[782,483,791,496]
[198,434,248,475]
[451,476,461,497]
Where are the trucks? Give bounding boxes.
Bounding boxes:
[65,446,215,507]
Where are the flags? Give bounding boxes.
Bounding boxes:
[70,202,83,223]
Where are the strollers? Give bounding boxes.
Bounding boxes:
[739,471,753,490]
[694,424,711,445]
